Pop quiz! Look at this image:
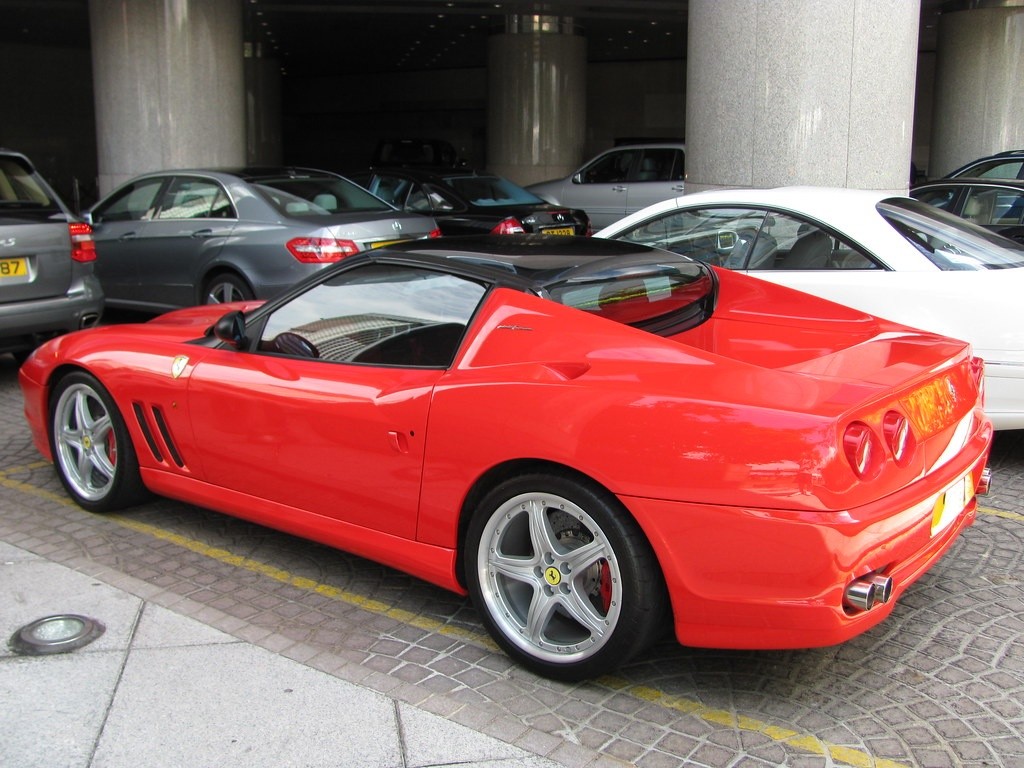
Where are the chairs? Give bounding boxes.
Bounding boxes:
[638,159,658,180]
[780,231,870,270]
[347,322,466,365]
[314,194,339,212]
[964,198,982,225]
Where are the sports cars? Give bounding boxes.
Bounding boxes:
[18,233,993,684]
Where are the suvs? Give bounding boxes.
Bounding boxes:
[0,147,106,368]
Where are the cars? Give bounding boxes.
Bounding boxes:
[82,164,443,315]
[304,158,592,238]
[908,148,1024,247]
[522,143,686,234]
[590,182,1024,433]
